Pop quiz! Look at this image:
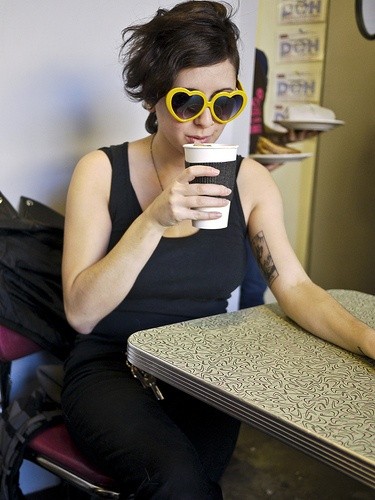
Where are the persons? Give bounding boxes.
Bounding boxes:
[249,48,326,173]
[61,0,375,500]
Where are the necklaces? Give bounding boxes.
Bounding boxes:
[150,132,164,192]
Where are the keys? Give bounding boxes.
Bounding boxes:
[127,358,164,399]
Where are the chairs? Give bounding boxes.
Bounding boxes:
[0,326,132,500]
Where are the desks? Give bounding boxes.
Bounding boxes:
[127,289,375,486]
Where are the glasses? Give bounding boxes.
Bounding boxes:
[165,78,248,123]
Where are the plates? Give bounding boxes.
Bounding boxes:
[273,120,347,131]
[251,152,313,162]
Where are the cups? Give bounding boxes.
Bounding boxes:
[182,143,238,229]
